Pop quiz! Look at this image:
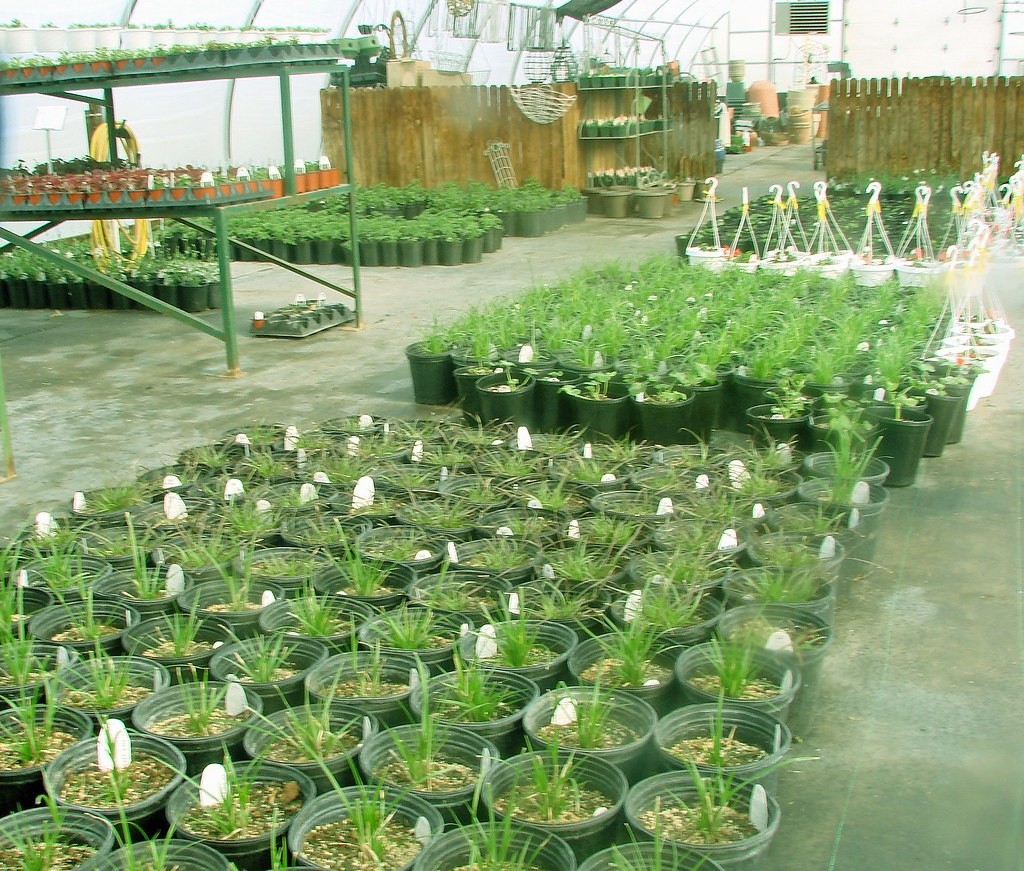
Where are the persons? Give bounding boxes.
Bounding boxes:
[714,98,731,147]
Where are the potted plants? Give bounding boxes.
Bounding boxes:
[80,525,164,570]
[29,588,142,659]
[615,759,780,871]
[88,839,240,871]
[286,755,444,871]
[27,539,114,606]
[0,619,80,708]
[120,613,233,685]
[485,748,628,864]
[45,730,187,844]
[0,545,34,587]
[49,634,170,735]
[164,760,317,871]
[131,681,265,778]
[412,771,577,871]
[72,482,153,523]
[152,488,893,777]
[0,560,55,644]
[90,567,193,622]
[24,512,97,555]
[577,842,727,871]
[0,806,115,871]
[0,0,1024,485]
[0,705,94,815]
